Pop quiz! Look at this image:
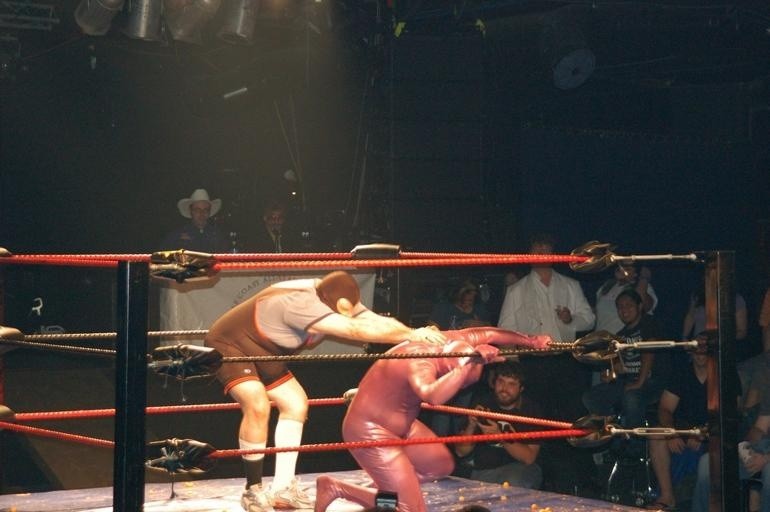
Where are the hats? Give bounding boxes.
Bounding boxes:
[177,189,222,219]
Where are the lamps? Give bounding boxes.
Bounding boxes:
[74,0,261,45]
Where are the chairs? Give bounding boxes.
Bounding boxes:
[600,356,672,507]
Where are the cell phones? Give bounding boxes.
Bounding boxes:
[375,490,398,512]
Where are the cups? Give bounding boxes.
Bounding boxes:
[736,440,760,477]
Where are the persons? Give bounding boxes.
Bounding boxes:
[313,326,552,512]
[427,232,769,510]
[204,270,447,512]
[242,200,310,254]
[152,189,244,255]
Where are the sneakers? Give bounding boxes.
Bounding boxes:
[242,482,275,512]
[266,478,316,509]
